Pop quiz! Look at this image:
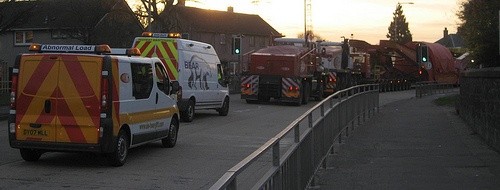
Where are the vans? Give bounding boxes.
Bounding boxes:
[7,43,184,168]
[130,31,231,122]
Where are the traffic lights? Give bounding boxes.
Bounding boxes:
[421,44,428,63]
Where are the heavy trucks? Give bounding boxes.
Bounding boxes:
[238,38,456,106]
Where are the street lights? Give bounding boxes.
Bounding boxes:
[394,1,415,42]
